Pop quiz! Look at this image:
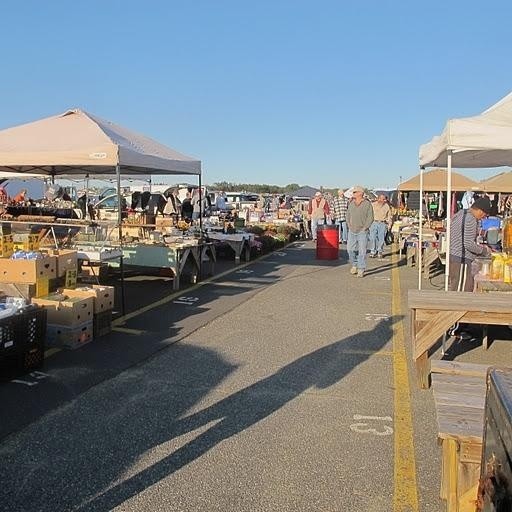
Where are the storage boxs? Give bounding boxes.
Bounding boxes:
[1,249,114,351]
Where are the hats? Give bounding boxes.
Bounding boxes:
[315,192,321,197]
[474,198,490,214]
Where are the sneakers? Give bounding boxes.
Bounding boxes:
[350,267,364,278]
[370,252,382,258]
[339,240,347,245]
[450,326,472,340]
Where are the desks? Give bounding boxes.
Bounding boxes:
[408,291,511,511]
[205,232,251,264]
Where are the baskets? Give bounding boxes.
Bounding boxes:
[0,309,53,366]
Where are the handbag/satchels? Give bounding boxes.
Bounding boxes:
[384,229,394,245]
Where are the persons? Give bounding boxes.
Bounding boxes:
[451,196,493,291]
[216,190,227,211]
[190,190,210,221]
[307,184,395,278]
[166,190,184,216]
[14,187,29,204]
[182,192,194,223]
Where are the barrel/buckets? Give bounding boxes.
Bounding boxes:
[317,224,339,261]
